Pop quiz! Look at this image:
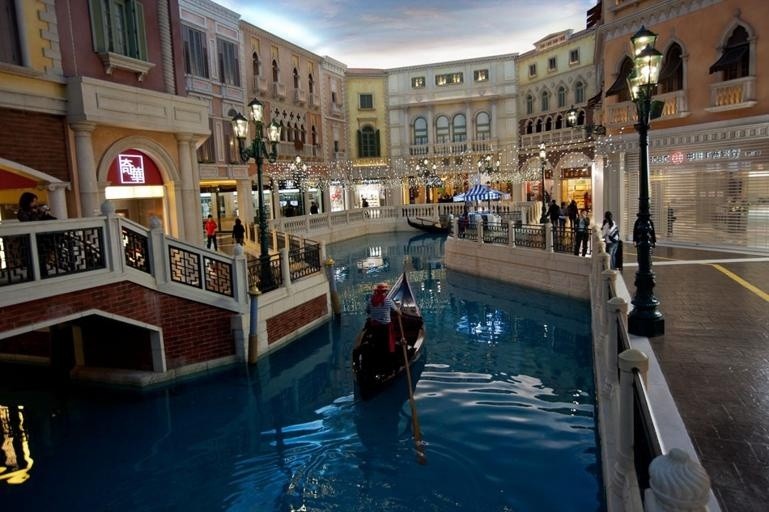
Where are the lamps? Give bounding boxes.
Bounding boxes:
[566,105,606,135]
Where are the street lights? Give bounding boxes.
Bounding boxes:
[230,98,283,293]
[477,154,500,213]
[415,158,437,203]
[288,155,308,215]
[539,142,549,223]
[624,25,666,336]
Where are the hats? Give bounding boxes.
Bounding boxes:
[373,283,390,292]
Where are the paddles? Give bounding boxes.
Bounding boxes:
[397,313,426,464]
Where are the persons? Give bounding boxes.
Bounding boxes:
[232,218,245,246]
[437,190,457,202]
[285,205,294,217]
[545,192,591,257]
[448,211,454,223]
[361,198,368,215]
[17,192,49,282]
[601,211,619,269]
[364,282,402,366]
[204,215,218,251]
[530,192,537,202]
[310,202,318,214]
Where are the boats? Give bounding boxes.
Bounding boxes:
[406,213,523,235]
[352,271,426,400]
